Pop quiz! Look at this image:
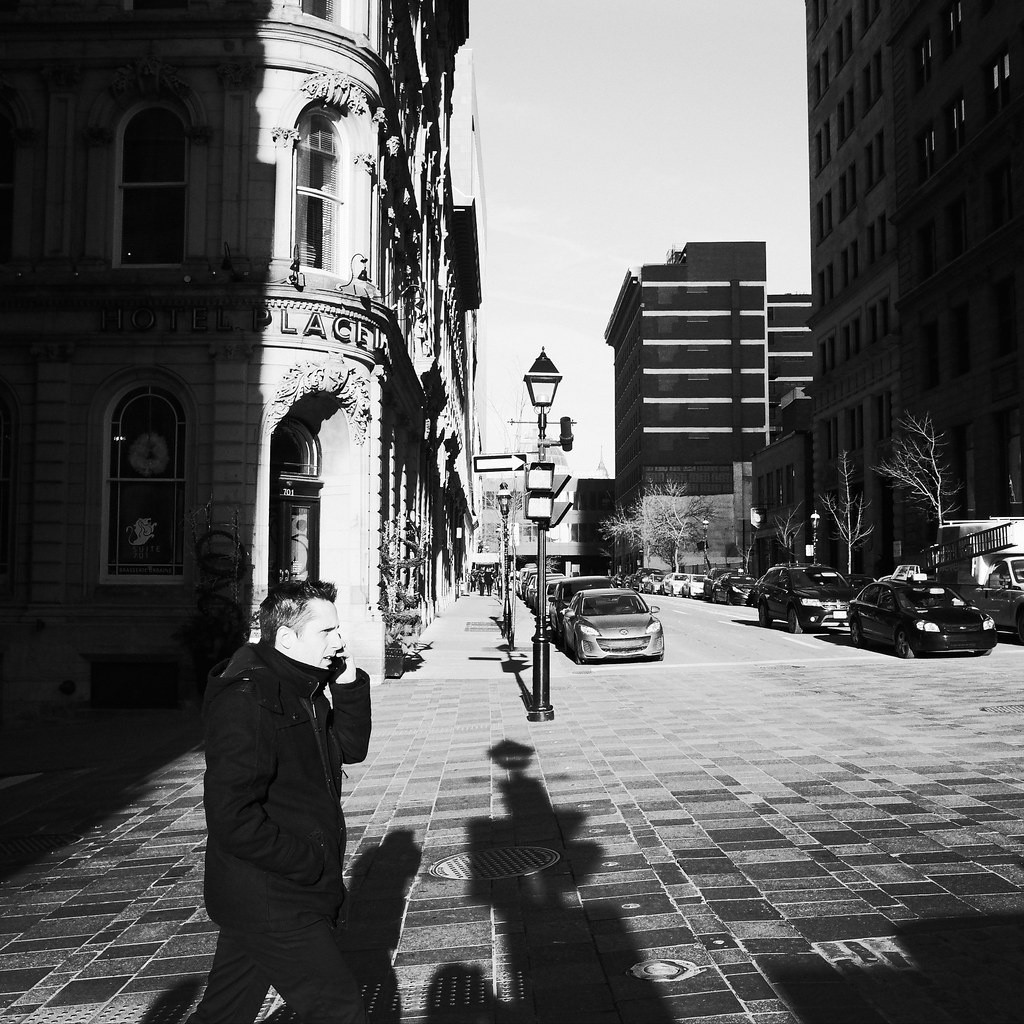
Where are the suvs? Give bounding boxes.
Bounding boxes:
[753,561,856,634]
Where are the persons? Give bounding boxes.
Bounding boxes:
[185,582,373,1024]
[466,566,503,598]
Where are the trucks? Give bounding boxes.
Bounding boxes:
[938,516,1024,645]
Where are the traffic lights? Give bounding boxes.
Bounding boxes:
[524,460,555,521]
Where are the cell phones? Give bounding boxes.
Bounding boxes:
[328,632,347,685]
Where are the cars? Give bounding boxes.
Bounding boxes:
[563,588,665,666]
[660,573,696,597]
[547,576,622,640]
[702,568,746,602]
[712,572,759,606]
[846,564,997,659]
[682,574,710,599]
[612,567,665,590]
[643,574,666,594]
[510,563,568,617]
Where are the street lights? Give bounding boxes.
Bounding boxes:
[496,482,514,652]
[702,518,709,575]
[811,509,820,564]
[522,346,564,725]
